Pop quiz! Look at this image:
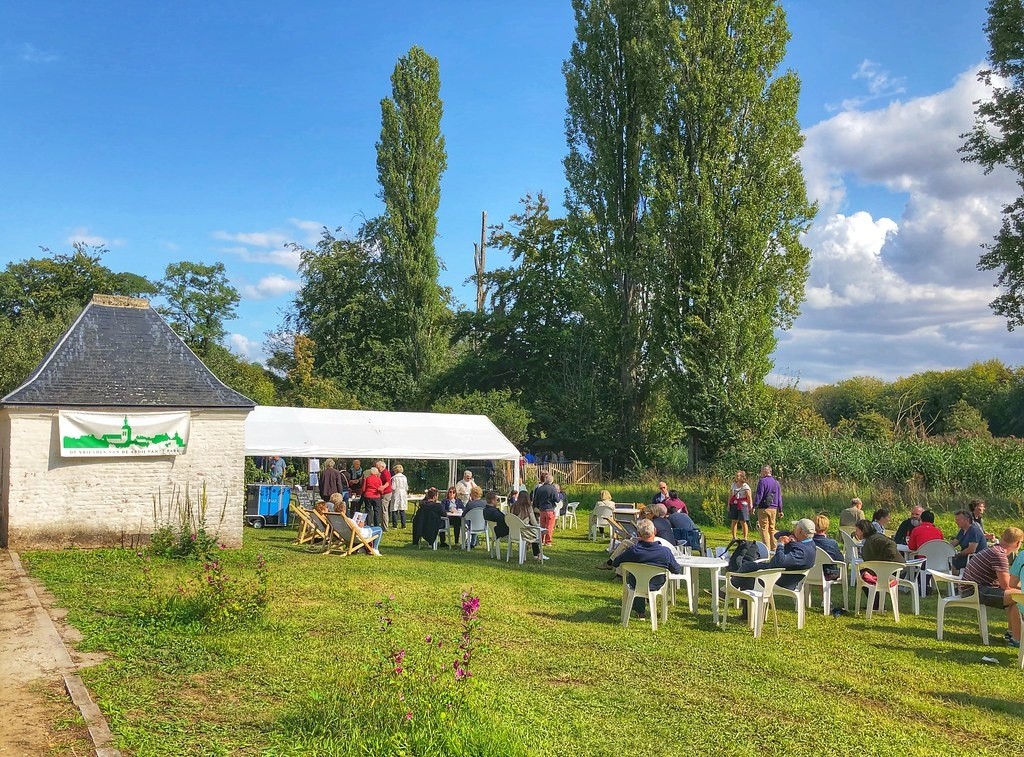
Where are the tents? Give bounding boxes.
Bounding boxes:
[245,405,520,498]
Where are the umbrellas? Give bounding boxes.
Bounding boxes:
[521,437,571,460]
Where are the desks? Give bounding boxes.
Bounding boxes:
[672,556,729,625]
[614,509,641,538]
[894,542,919,594]
[406,494,425,522]
[445,509,469,549]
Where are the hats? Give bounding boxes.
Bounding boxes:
[791,518,816,534]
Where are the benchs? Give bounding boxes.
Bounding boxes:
[289,503,380,557]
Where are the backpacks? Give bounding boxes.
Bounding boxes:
[718,538,762,572]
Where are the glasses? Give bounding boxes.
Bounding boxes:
[660,486,665,489]
[450,491,455,494]
[466,477,472,480]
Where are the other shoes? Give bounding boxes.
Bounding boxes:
[534,553,550,560]
[733,614,748,621]
[373,549,382,556]
[703,589,725,601]
[596,562,613,571]
[1004,631,1020,647]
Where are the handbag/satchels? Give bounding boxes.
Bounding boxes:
[758,493,774,508]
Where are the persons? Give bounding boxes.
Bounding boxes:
[752,465,784,554]
[958,527,1024,648]
[703,518,816,621]
[948,498,996,596]
[840,497,943,612]
[727,470,752,542]
[420,470,568,559]
[594,504,677,580]
[813,514,845,608]
[269,455,286,485]
[315,458,409,556]
[613,518,682,618]
[594,489,616,537]
[624,482,695,529]
[482,449,567,491]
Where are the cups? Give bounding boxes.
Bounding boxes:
[685,546,692,559]
[677,545,684,554]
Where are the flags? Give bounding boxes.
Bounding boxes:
[58,410,190,457]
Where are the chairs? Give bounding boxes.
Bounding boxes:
[619,525,1003,654]
[589,506,613,541]
[504,513,543,564]
[497,495,508,511]
[418,517,451,550]
[563,502,580,530]
[462,507,502,559]
